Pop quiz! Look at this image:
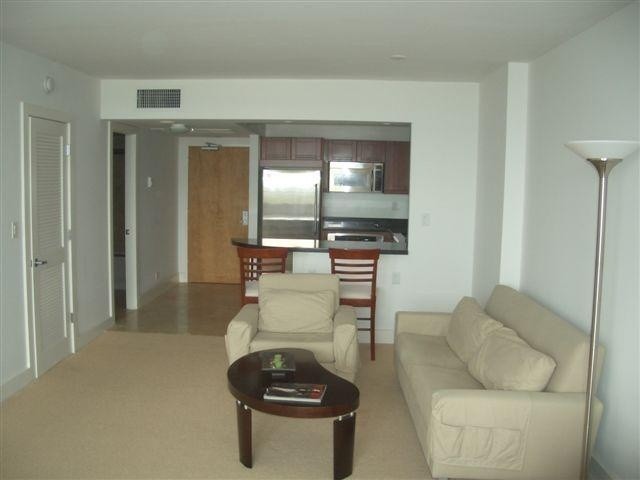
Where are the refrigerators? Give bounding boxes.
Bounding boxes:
[260,167,320,238]
[262,239,321,249]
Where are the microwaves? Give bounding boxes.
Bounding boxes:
[327,162,382,194]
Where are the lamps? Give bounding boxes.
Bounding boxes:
[562,138,640,480]
[169,123,188,134]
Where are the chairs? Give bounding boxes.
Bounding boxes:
[222,248,381,384]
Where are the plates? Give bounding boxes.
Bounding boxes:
[260,355,299,376]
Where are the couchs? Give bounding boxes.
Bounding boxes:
[392,283,605,480]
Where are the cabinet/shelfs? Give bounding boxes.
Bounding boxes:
[384,140,409,192]
[258,135,322,164]
[322,139,384,162]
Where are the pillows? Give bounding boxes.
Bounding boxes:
[442,294,556,392]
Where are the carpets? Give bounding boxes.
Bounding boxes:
[0,328,431,480]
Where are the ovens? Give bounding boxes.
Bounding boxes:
[327,234,383,242]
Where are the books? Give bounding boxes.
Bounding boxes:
[262,381,329,404]
